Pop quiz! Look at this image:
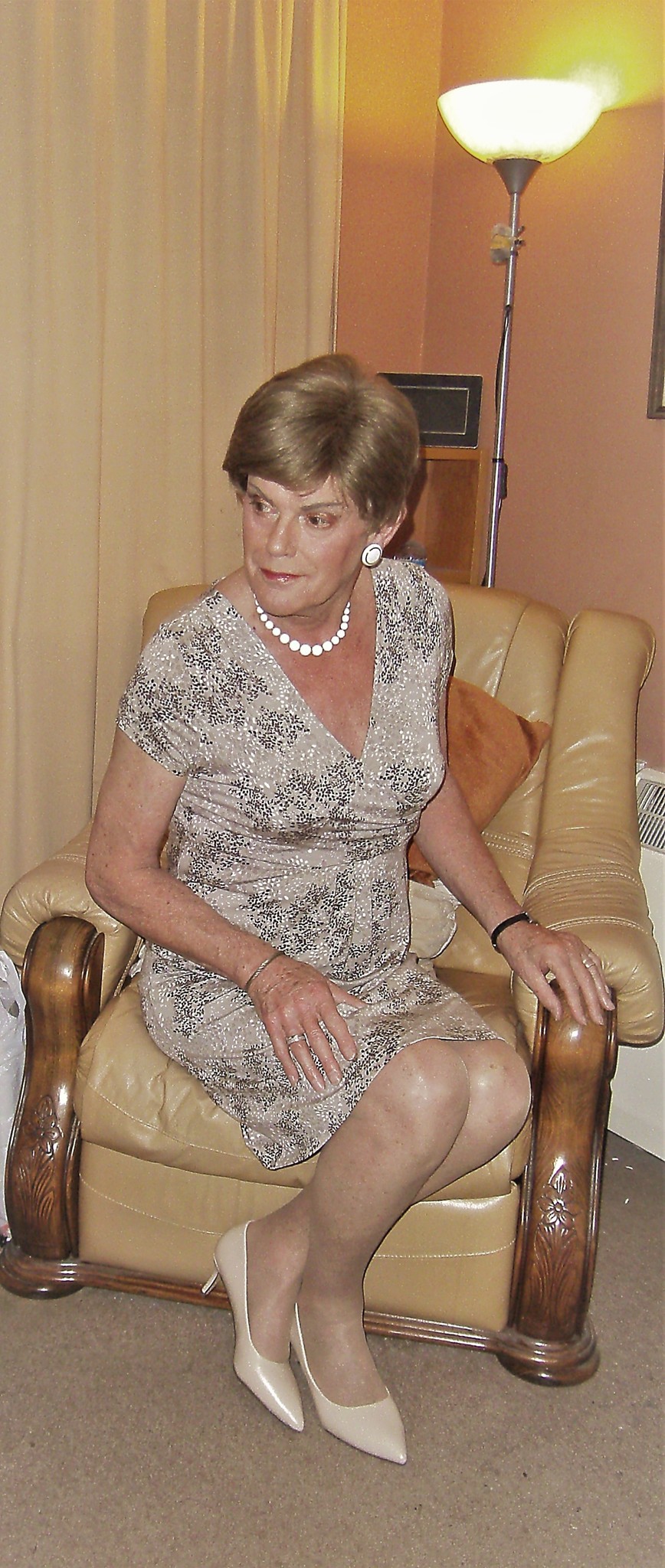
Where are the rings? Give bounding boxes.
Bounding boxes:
[583,958,595,968]
[588,951,593,957]
[286,1034,306,1045]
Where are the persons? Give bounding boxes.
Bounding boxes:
[86,351,615,1463]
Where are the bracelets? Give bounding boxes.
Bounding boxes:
[244,952,284,991]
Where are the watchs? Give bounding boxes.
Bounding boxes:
[492,911,537,955]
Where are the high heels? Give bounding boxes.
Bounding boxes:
[201,1220,304,1434]
[289,1302,407,1466]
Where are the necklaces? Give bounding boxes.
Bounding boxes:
[253,593,351,656]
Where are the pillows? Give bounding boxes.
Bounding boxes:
[408,678,554,892]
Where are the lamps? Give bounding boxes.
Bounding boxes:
[430,74,617,597]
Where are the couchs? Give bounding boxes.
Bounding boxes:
[0,576,665,1394]
